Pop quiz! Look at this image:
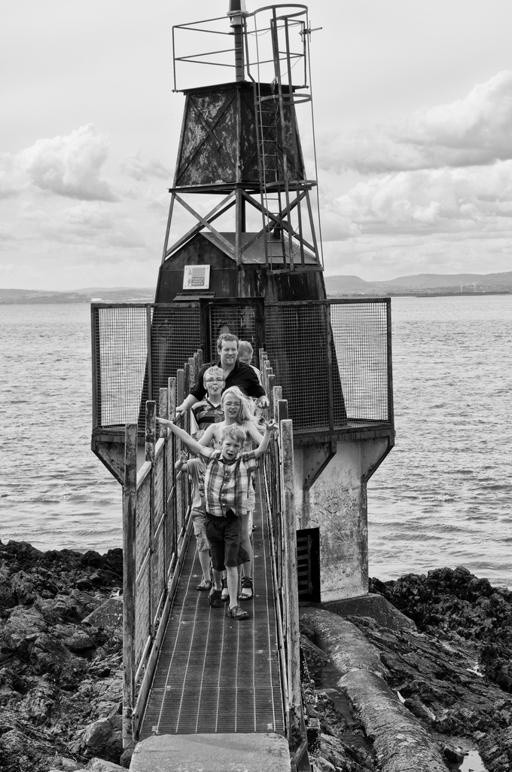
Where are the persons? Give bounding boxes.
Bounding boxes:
[154,333,280,621]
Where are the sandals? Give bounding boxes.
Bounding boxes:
[195,577,253,619]
